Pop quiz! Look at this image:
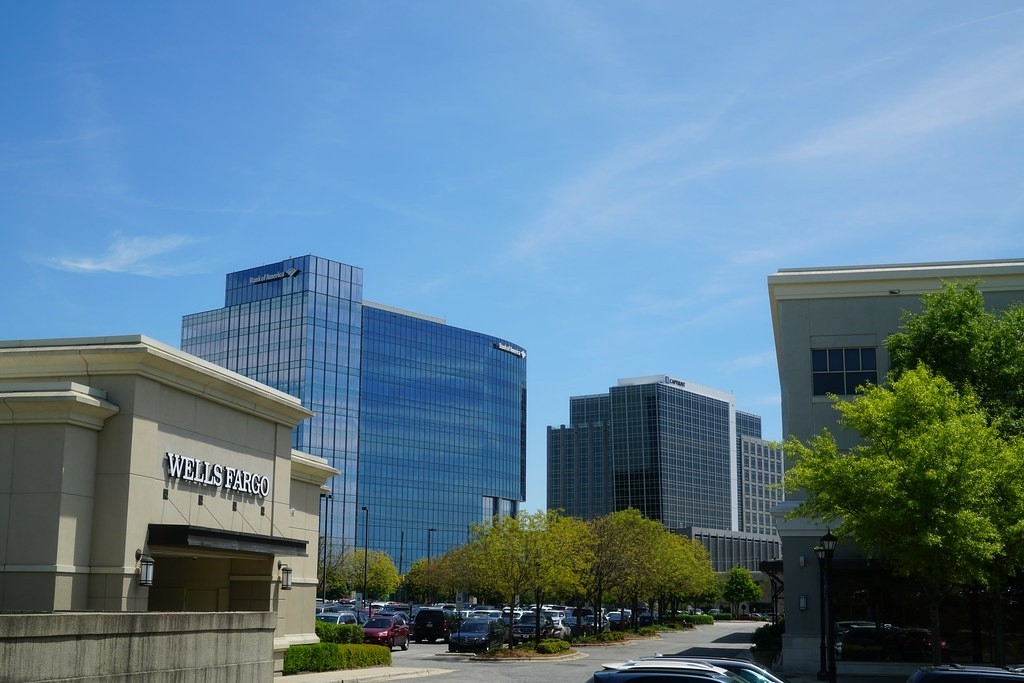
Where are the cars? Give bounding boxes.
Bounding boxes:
[594,652,793,683]
[546,619,572,639]
[907,663,1024,683]
[316,612,358,627]
[752,613,761,617]
[361,617,410,651]
[373,611,413,636]
[640,614,658,627]
[586,615,610,634]
[693,609,703,615]
[739,614,751,620]
[708,609,723,614]
[840,625,950,665]
[449,617,505,653]
[316,598,648,626]
[563,617,592,638]
[610,615,633,629]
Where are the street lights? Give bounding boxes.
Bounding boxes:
[820,527,839,683]
[814,543,829,682]
[323,494,334,605]
[319,493,326,564]
[361,506,369,607]
[427,528,436,562]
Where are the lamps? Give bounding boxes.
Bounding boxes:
[277,561,292,590]
[135,549,154,587]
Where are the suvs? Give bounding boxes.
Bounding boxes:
[414,607,456,644]
[508,613,554,644]
